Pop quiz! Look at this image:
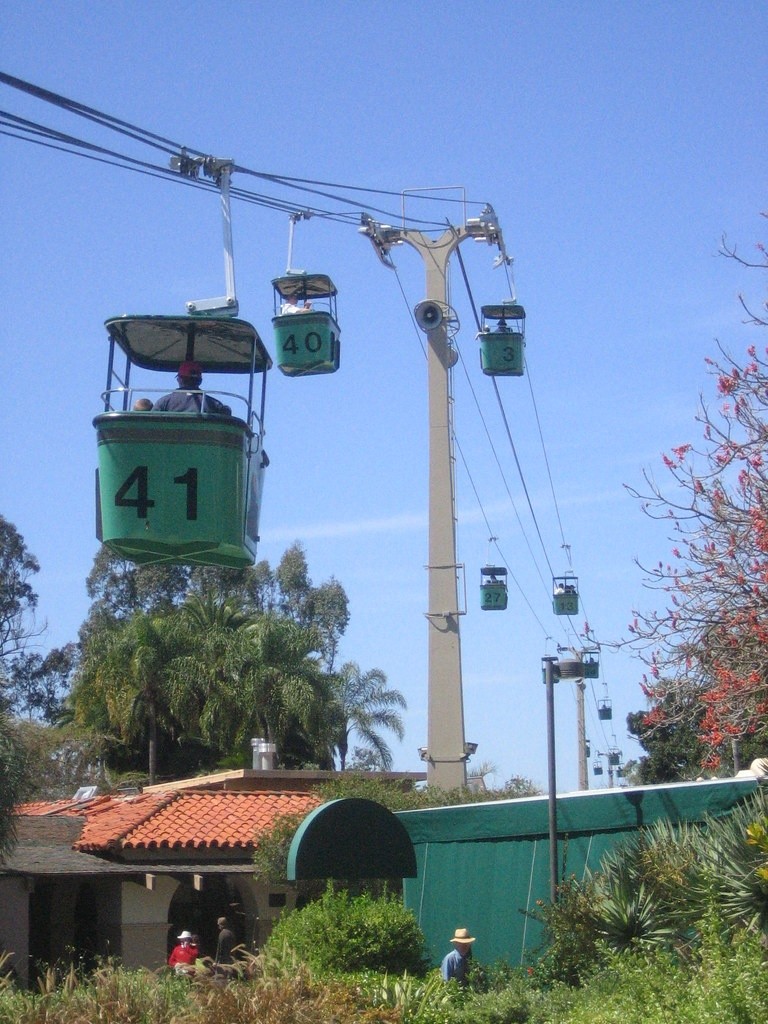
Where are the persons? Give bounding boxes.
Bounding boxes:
[442,928,476,987]
[134,361,232,415]
[494,319,513,333]
[216,917,234,964]
[487,574,504,584]
[169,931,200,975]
[555,583,577,596]
[282,292,316,316]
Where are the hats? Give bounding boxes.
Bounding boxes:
[450,929,475,943]
[497,318,507,326]
[177,931,192,939]
[173,361,202,379]
[134,398,153,412]
[484,324,490,332]
[217,917,227,924]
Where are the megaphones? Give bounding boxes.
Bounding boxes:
[416,301,443,330]
